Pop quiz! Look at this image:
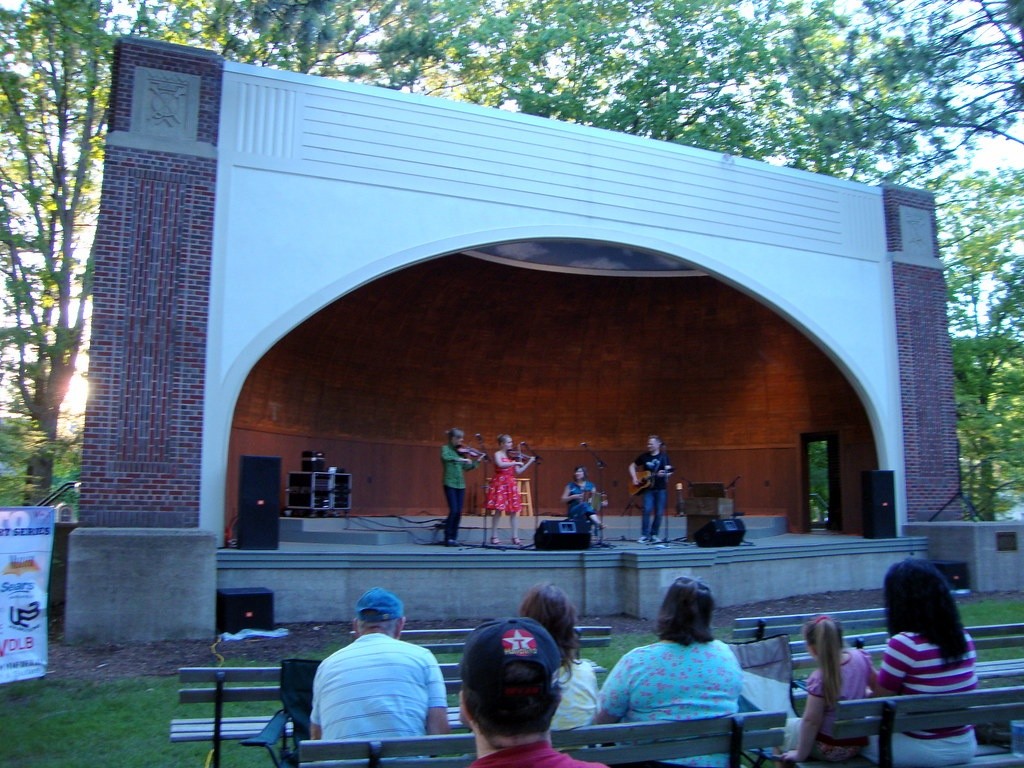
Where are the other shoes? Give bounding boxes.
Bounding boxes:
[445,539,462,547]
[636,536,650,543]
[512,537,523,546]
[491,536,501,545]
[598,522,608,530]
[651,537,661,542]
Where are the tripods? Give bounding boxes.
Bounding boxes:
[585,446,618,549]
[519,446,544,550]
[648,447,690,545]
[466,436,500,549]
[928,443,982,522]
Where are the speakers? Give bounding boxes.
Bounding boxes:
[535,519,591,549]
[217,588,274,634]
[928,560,967,589]
[236,455,283,549]
[695,518,745,547]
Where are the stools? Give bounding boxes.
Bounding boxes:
[481,478,533,516]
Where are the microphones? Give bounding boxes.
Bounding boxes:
[475,433,482,439]
[580,443,587,446]
[520,442,528,446]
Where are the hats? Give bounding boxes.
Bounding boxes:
[460,616,563,695]
[354,586,404,622]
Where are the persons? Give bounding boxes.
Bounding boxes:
[458,615,610,768]
[770,615,883,768]
[861,557,977,768]
[519,581,602,752]
[592,576,743,768]
[308,587,451,761]
[483,433,536,546]
[629,435,673,544]
[561,464,609,543]
[439,428,486,547]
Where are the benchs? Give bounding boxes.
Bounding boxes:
[170,608,1024,768]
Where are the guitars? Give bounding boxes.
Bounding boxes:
[626,465,677,497]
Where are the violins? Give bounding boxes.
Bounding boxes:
[506,448,542,465]
[456,444,490,460]
